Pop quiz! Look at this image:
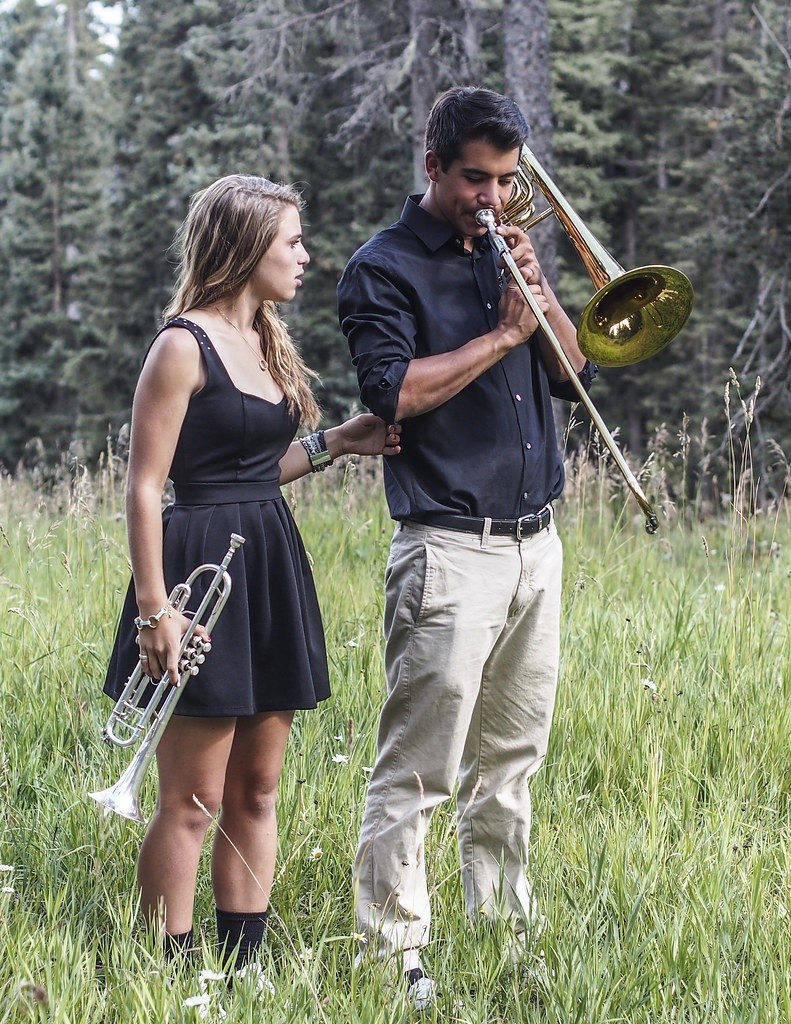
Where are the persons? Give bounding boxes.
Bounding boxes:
[336,83,595,1012]
[101,172,403,1000]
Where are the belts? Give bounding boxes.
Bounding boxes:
[404,504,556,537]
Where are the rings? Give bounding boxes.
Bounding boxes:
[139,655,148,662]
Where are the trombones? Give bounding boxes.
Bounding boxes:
[477,144,695,537]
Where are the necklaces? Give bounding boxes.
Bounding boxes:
[212,302,269,372]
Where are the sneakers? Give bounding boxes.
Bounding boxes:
[356,949,441,1008]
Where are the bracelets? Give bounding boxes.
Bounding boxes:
[134,599,173,631]
[300,427,335,474]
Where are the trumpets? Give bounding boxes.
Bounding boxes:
[88,530,247,821]
